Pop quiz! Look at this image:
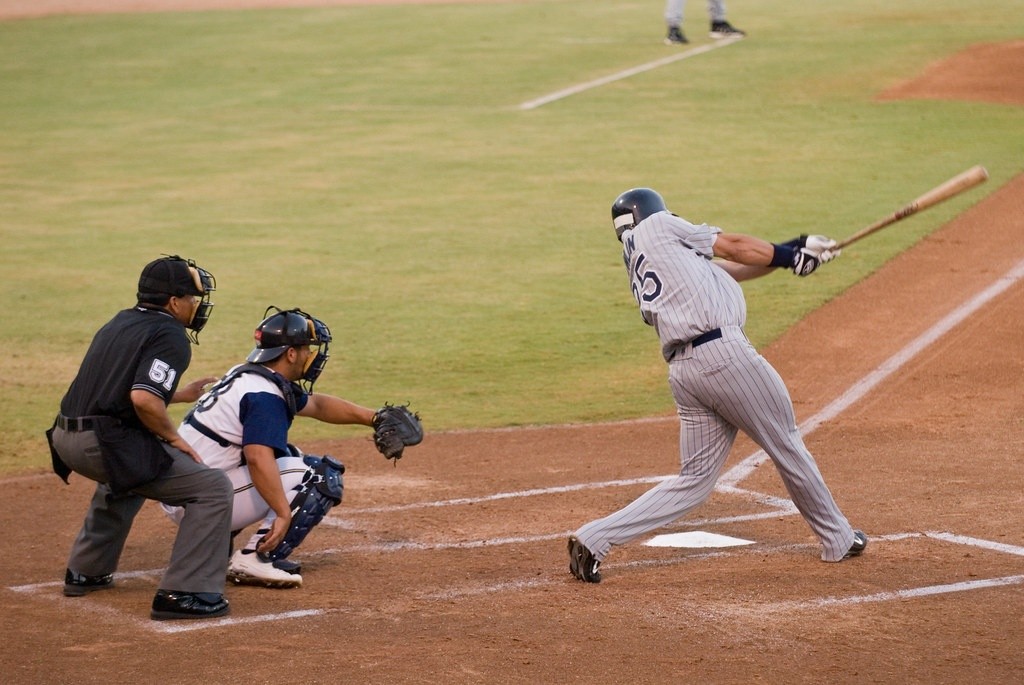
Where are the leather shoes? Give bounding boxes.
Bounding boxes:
[151,590,229,620]
[63,569,113,597]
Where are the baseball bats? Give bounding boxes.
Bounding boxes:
[829,164,989,252]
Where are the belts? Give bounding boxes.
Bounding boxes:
[56,416,93,431]
[670,328,722,357]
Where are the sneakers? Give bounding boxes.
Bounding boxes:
[568,535,601,583]
[838,529,866,557]
[226,549,302,588]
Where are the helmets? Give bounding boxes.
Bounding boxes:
[611,188,677,243]
[246,311,311,362]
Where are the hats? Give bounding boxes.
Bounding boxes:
[139,255,208,296]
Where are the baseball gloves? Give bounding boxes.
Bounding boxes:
[365,400,425,469]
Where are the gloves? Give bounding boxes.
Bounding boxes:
[770,235,841,277]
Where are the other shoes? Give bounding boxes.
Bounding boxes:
[710,21,745,38]
[665,30,688,46]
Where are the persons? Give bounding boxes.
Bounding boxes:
[566,188,868,585]
[159,307,423,590]
[44,254,234,618]
[663,0,746,46]
